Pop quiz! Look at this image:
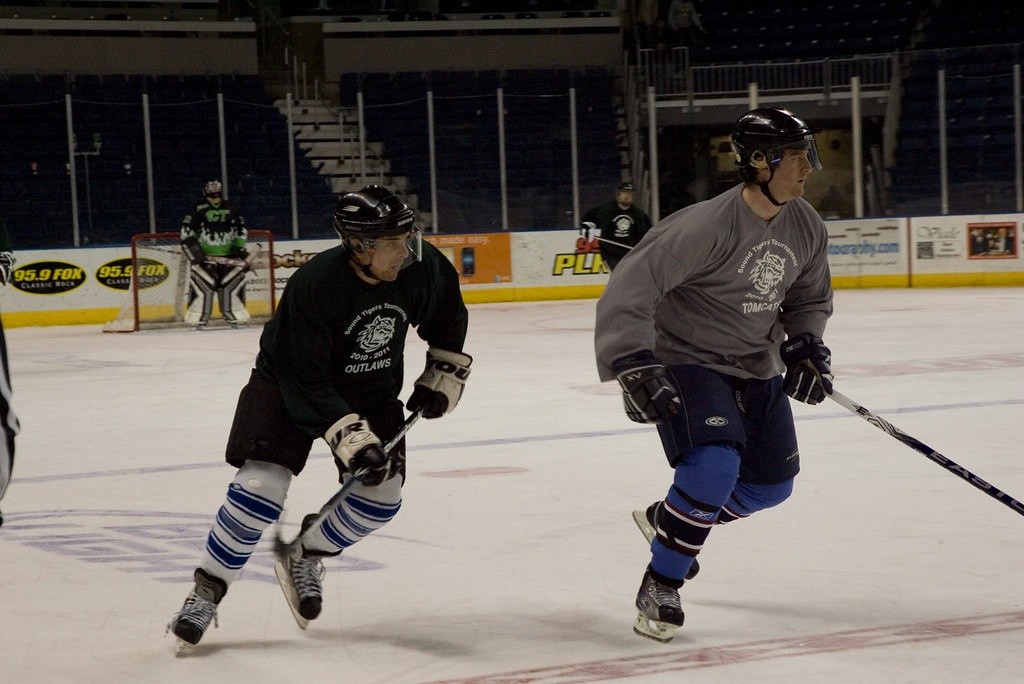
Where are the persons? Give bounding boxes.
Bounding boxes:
[594,106,834,645]
[161,183,474,660]
[179,179,252,331]
[579,182,652,271]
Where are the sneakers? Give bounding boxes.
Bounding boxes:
[632,513,699,582]
[165,566,228,656]
[274,513,343,630]
[633,563,684,642]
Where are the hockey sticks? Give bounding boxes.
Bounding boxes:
[137,244,259,280]
[271,404,425,566]
[592,235,635,251]
[818,376,1024,518]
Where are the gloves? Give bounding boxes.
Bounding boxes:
[583,228,595,241]
[323,415,390,486]
[779,332,834,405]
[611,349,681,423]
[0,252,16,286]
[406,345,472,418]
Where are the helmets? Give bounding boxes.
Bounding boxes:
[731,107,814,167]
[617,182,635,192]
[331,184,415,249]
[205,181,223,195]
